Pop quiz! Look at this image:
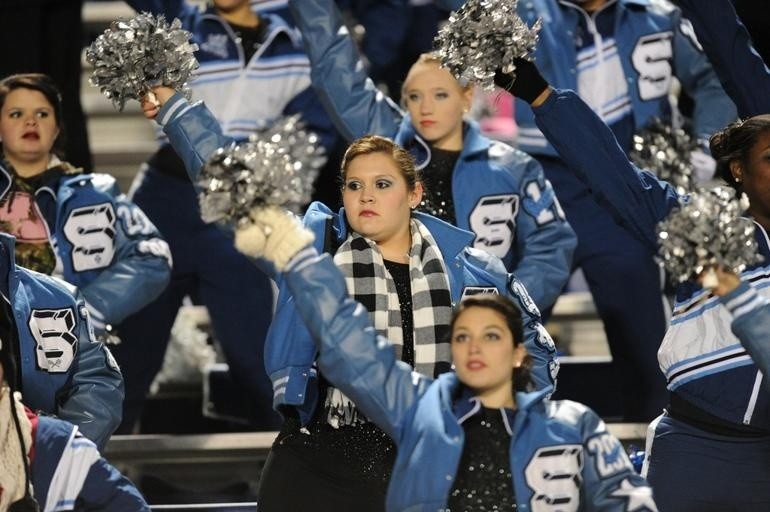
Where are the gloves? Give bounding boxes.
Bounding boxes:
[232,205,317,273]
[494,57,549,105]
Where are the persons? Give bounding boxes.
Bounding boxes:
[2,1,769,511]
